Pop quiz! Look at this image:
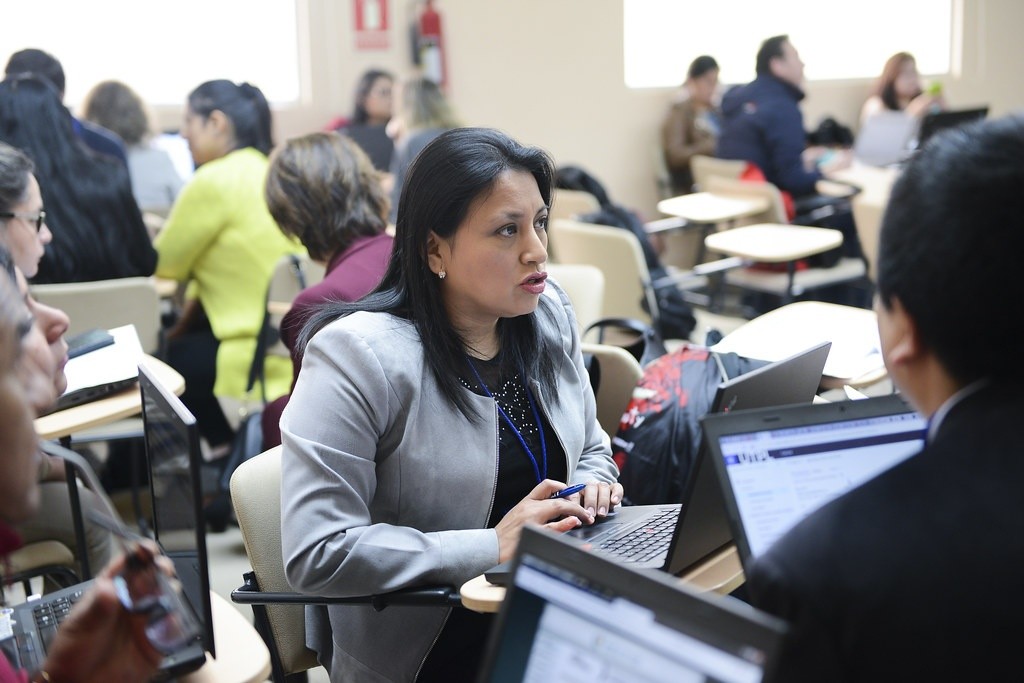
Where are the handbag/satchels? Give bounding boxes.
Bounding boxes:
[580,317,668,370]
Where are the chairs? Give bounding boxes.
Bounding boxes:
[686,154,867,310]
[228,445,466,683]
[546,156,654,435]
[29,278,159,540]
[265,249,328,321]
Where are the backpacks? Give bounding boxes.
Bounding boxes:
[554,166,614,213]
[582,207,697,341]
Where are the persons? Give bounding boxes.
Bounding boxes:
[713,35,869,302]
[149,79,309,500]
[253,130,395,682]
[80,79,182,217]
[0,48,158,285]
[663,56,717,195]
[386,78,462,226]
[0,141,183,683]
[279,128,624,683]
[854,52,949,168]
[751,113,1024,683]
[319,69,395,172]
[552,166,697,341]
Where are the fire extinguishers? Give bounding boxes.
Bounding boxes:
[419,0,447,84]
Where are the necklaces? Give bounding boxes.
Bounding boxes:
[465,336,548,483]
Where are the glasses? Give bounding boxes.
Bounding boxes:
[1,211,47,232]
[81,509,207,654]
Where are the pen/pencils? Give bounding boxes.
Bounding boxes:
[550,484,586,499]
